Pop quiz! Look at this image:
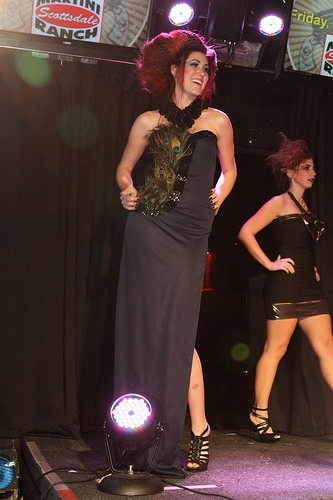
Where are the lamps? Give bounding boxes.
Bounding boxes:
[253,0,284,37]
[162,0,195,27]
[97,392,165,496]
[0,449,19,500]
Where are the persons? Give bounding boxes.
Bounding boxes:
[111,30,238,480]
[238,140,333,442]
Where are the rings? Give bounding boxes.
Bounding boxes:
[121,201,124,205]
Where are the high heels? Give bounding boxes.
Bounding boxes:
[247,406,282,442]
[185,423,211,471]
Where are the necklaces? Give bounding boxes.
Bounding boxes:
[301,199,306,209]
